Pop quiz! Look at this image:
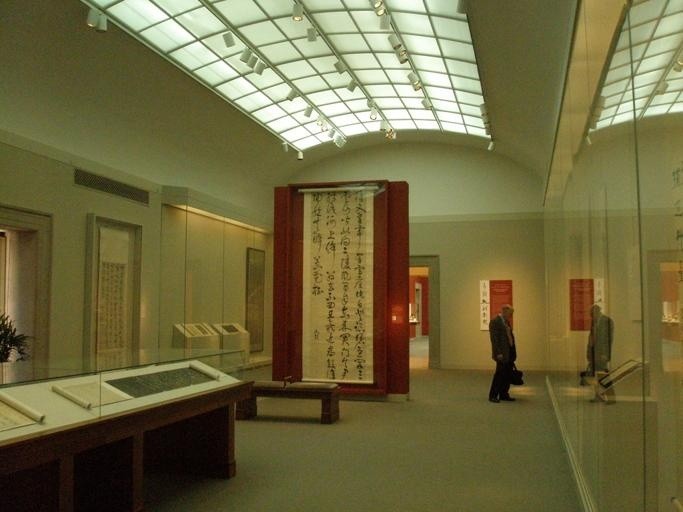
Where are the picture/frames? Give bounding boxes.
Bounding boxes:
[599,357,640,387]
[246,245,266,354]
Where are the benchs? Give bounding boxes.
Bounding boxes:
[236,381,341,425]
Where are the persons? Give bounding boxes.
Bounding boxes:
[489,303,517,403]
[586,304,616,405]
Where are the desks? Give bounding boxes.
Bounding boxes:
[0,359,256,512]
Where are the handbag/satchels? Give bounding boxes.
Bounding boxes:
[497,364,523,386]
[580,364,593,386]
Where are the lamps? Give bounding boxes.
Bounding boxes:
[478,101,496,152]
[86,7,108,32]
[220,0,433,161]
[585,95,608,148]
[652,82,670,97]
[670,53,683,69]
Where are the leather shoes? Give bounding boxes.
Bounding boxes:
[499,398,515,401]
[605,399,616,405]
[588,398,604,403]
[489,398,499,402]
